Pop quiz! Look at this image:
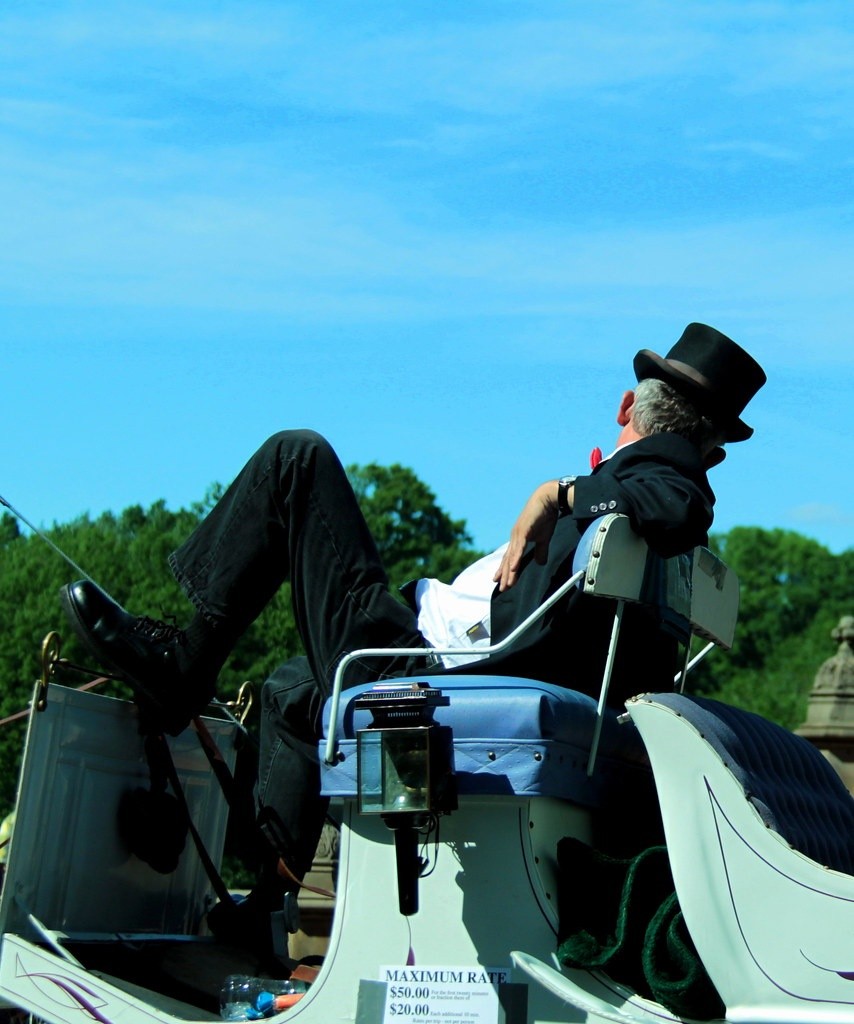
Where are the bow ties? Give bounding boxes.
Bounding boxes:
[590,447,603,470]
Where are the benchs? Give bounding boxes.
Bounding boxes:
[317,513,741,798]
[623,692,854,1024]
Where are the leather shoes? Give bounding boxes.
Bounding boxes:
[208,898,293,967]
[65,581,218,735]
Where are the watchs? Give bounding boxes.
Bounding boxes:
[557,475,577,515]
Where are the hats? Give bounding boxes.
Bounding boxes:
[634,322,768,442]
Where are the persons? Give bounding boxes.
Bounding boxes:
[61,324,767,965]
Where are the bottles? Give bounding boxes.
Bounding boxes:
[221,975,312,1024]
